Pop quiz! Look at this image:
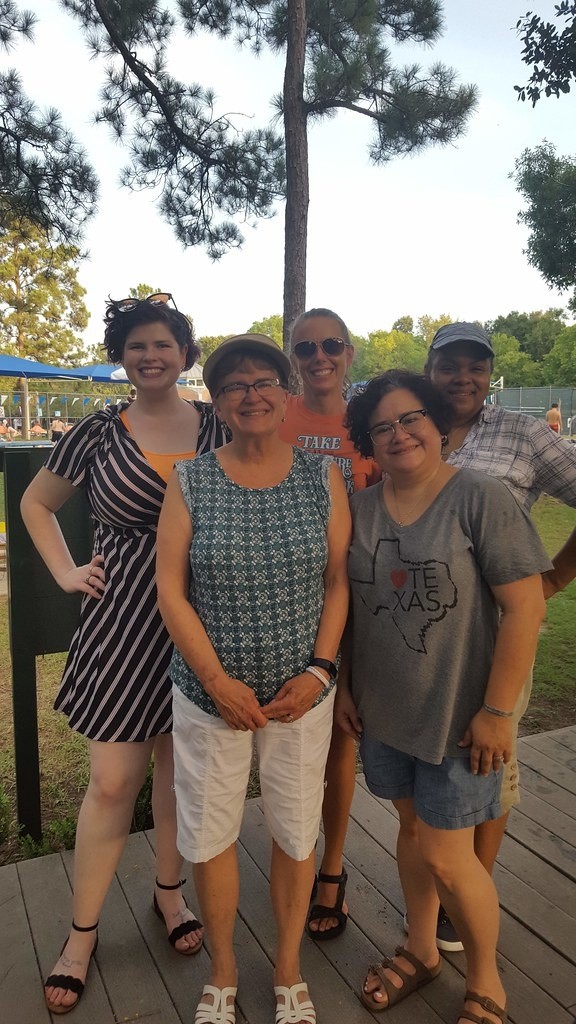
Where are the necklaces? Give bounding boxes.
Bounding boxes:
[391,483,426,528]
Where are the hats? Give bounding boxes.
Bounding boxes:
[202,334,292,390]
[430,321,494,359]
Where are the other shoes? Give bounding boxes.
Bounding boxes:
[403,904,465,951]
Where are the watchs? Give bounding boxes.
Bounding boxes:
[307,655,338,679]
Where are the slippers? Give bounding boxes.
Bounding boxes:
[361,944,443,1010]
[195,968,239,1024]
[456,987,508,1024]
[272,968,316,1024]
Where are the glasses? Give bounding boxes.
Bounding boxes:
[366,409,430,446]
[293,337,350,361]
[116,293,178,313]
[216,379,288,401]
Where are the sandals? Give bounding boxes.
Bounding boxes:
[306,866,349,942]
[153,876,205,956]
[44,919,100,1015]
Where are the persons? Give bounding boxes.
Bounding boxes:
[334,321,576,1024]
[156,333,353,1024]
[18,292,234,1014]
[545,403,563,433]
[275,308,387,942]
[0,416,74,448]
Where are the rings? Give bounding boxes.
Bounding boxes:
[289,715,294,719]
[89,566,95,574]
[493,756,503,761]
[86,574,92,585]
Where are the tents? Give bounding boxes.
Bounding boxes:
[0,352,190,385]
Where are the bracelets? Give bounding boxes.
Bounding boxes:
[482,704,513,718]
[305,666,331,690]
